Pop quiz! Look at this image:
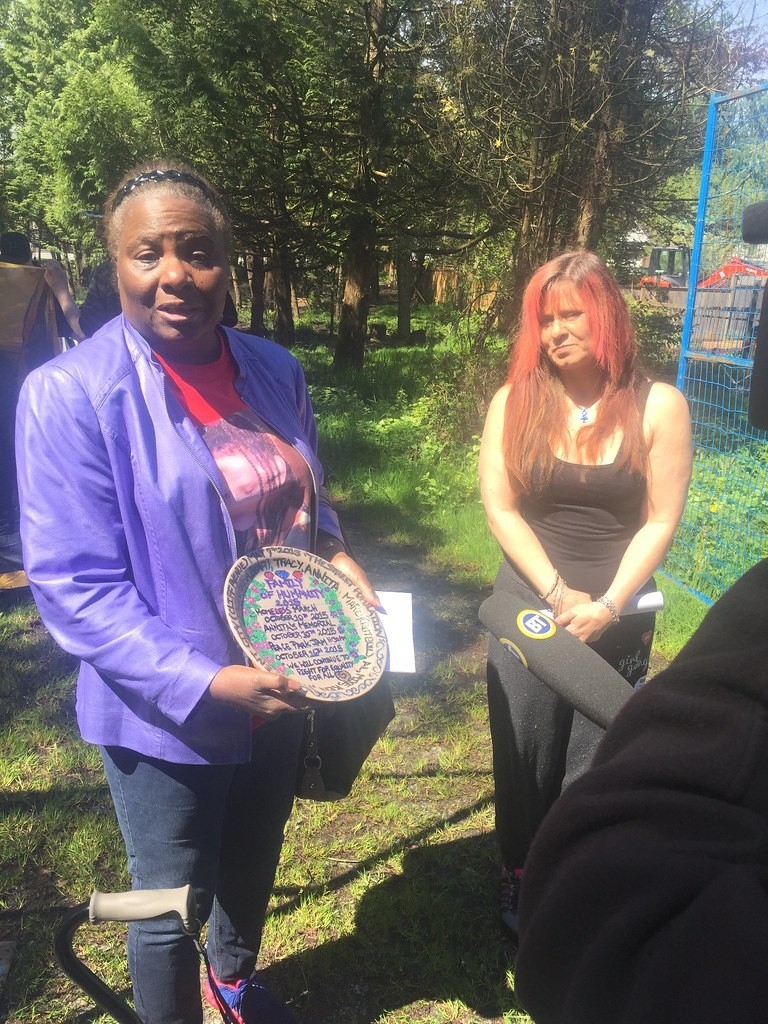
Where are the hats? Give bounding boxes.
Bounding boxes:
[0,232,31,264]
[84,202,106,219]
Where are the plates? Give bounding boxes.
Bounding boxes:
[223,547,389,703]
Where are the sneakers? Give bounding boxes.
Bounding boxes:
[204,962,294,1024]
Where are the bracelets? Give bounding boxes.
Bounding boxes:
[597,594,620,628]
[537,567,566,620]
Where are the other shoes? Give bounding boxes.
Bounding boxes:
[499,860,522,940]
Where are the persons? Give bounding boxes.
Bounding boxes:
[1,229,123,343]
[510,545,768,1023]
[475,251,694,923]
[12,160,388,1023]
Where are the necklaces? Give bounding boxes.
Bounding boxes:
[552,366,603,424]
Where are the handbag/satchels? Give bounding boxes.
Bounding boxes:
[291,676,396,802]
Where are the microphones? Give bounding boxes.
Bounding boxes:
[478,590,636,731]
[742,200,768,244]
